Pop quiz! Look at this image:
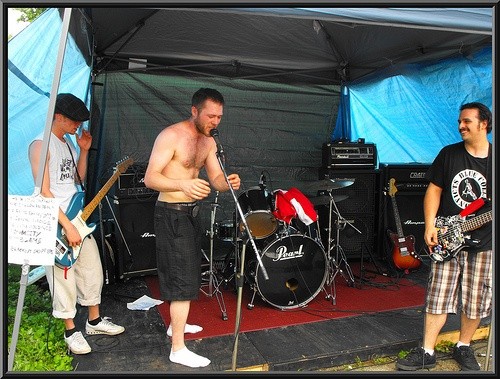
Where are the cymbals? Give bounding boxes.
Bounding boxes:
[301,178,356,191]
[308,196,350,206]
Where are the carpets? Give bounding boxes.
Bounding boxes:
[145,261,426,340]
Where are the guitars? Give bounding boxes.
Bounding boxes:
[54,156,134,271]
[388,178,422,273]
[429,210,493,264]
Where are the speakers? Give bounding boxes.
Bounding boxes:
[102,196,157,282]
[320,168,376,261]
[378,191,431,259]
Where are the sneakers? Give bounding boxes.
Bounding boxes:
[85,317,125,335]
[64,331,91,354]
[395,348,436,370]
[452,342,480,371]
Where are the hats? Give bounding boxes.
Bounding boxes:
[55,93,90,122]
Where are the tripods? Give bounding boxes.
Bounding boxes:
[197,188,229,321]
[308,195,381,305]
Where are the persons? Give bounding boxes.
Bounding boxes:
[28,93,125,354]
[395,101,492,371]
[143,88,241,369]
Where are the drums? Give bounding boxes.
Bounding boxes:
[237,186,280,239]
[216,220,243,242]
[243,223,329,311]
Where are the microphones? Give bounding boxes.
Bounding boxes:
[258,171,264,189]
[210,128,224,154]
[319,189,332,195]
